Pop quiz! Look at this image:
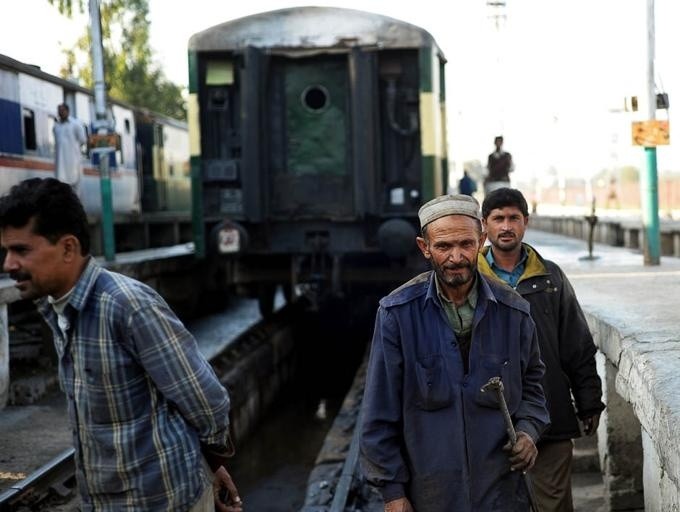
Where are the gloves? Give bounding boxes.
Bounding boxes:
[503,432,538,471]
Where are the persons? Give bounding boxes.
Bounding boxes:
[359,194,551,510]
[53,104,84,185]
[481,188,605,511]
[0,177,243,512]
[485,136,514,188]
[459,170,476,195]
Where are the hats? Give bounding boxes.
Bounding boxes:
[417,194,482,230]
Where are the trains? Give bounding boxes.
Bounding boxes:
[188,7,448,320]
[0,52,188,276]
